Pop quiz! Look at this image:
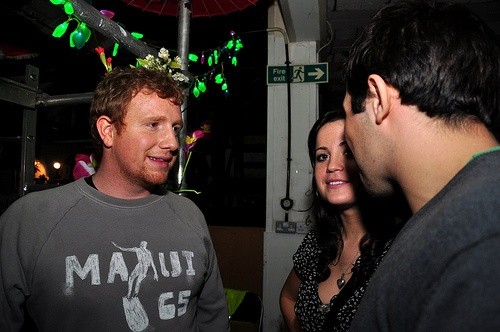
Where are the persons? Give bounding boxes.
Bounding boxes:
[0,67,230,332]
[343,0,500,332]
[279,108,407,332]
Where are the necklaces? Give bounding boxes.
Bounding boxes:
[337,251,359,289]
